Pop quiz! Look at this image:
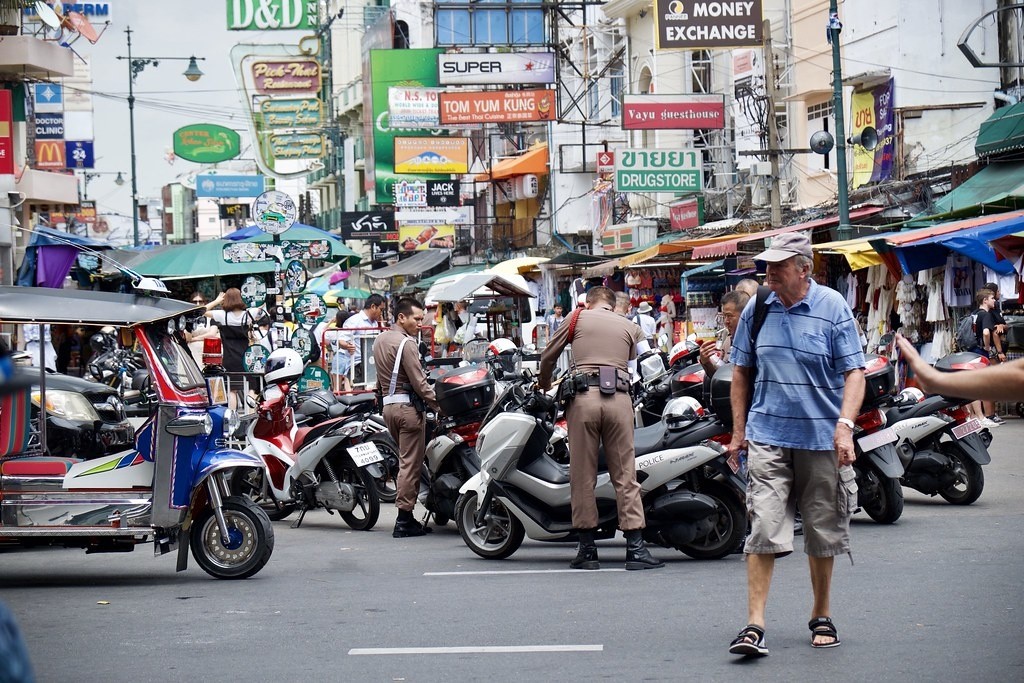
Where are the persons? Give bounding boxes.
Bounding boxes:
[205,288,266,413]
[374,298,445,538]
[538,286,665,570]
[895,332,1024,402]
[656,300,676,353]
[325,294,386,396]
[728,233,867,656]
[970,283,1009,428]
[701,279,760,380]
[633,302,657,348]
[178,291,218,387]
[456,300,478,334]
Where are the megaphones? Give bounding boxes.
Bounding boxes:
[810,131,834,154]
[847,126,879,151]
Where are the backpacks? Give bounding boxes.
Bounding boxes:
[955,314,978,352]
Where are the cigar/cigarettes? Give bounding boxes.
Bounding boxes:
[717,326,728,333]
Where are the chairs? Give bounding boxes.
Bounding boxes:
[0,381,85,478]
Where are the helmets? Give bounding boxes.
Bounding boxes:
[668,340,700,367]
[264,348,303,384]
[90,326,119,352]
[661,396,705,430]
[488,337,519,358]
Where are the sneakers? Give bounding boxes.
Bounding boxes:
[979,417,999,428]
[987,415,1006,425]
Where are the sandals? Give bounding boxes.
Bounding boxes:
[808,616,841,648]
[728,624,769,658]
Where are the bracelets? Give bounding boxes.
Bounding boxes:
[838,418,854,429]
[998,351,1002,354]
[700,360,710,366]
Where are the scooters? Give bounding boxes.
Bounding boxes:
[9,282,994,564]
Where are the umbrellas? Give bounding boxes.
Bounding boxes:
[494,257,551,274]
[133,222,361,278]
[333,288,372,305]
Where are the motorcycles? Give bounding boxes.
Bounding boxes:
[0,277,276,581]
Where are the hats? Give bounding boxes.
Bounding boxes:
[751,233,814,262]
[661,294,674,305]
[636,302,652,313]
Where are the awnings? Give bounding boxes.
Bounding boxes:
[830,71,891,88]
[474,146,550,181]
[365,249,449,280]
[781,89,834,102]
[582,208,1024,278]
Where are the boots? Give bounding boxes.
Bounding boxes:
[391,508,427,538]
[569,528,600,570]
[622,530,665,570]
[411,511,433,533]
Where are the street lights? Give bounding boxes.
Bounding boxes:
[114,25,207,248]
[77,168,128,238]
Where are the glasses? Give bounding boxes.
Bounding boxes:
[191,300,204,305]
